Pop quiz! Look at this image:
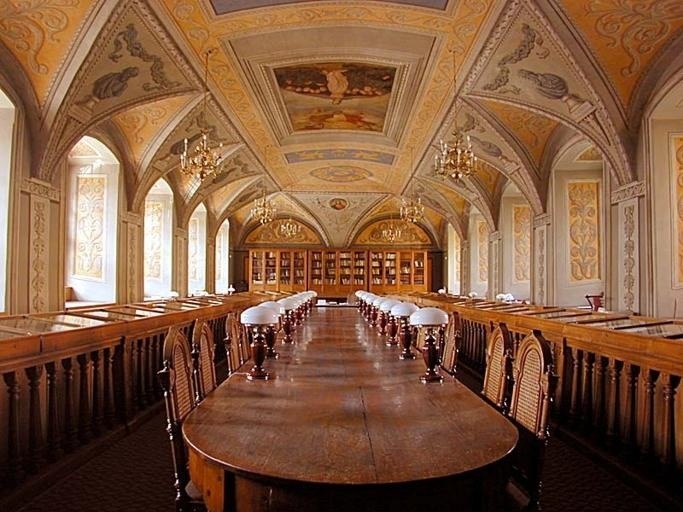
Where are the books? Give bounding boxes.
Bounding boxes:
[253,252,423,285]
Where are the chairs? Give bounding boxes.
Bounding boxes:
[508,330,555,502]
[161,325,201,504]
[225,312,243,374]
[479,321,511,409]
[190,317,219,395]
[416,314,435,353]
[439,310,461,373]
[235,307,251,361]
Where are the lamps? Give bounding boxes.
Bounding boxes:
[180,53,223,184]
[401,149,425,223]
[354,291,450,384]
[251,144,278,225]
[240,289,318,382]
[381,198,403,242]
[279,186,302,238]
[434,52,479,183]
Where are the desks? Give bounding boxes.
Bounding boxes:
[179,290,520,511]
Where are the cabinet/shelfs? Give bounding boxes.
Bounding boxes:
[249,248,428,299]
